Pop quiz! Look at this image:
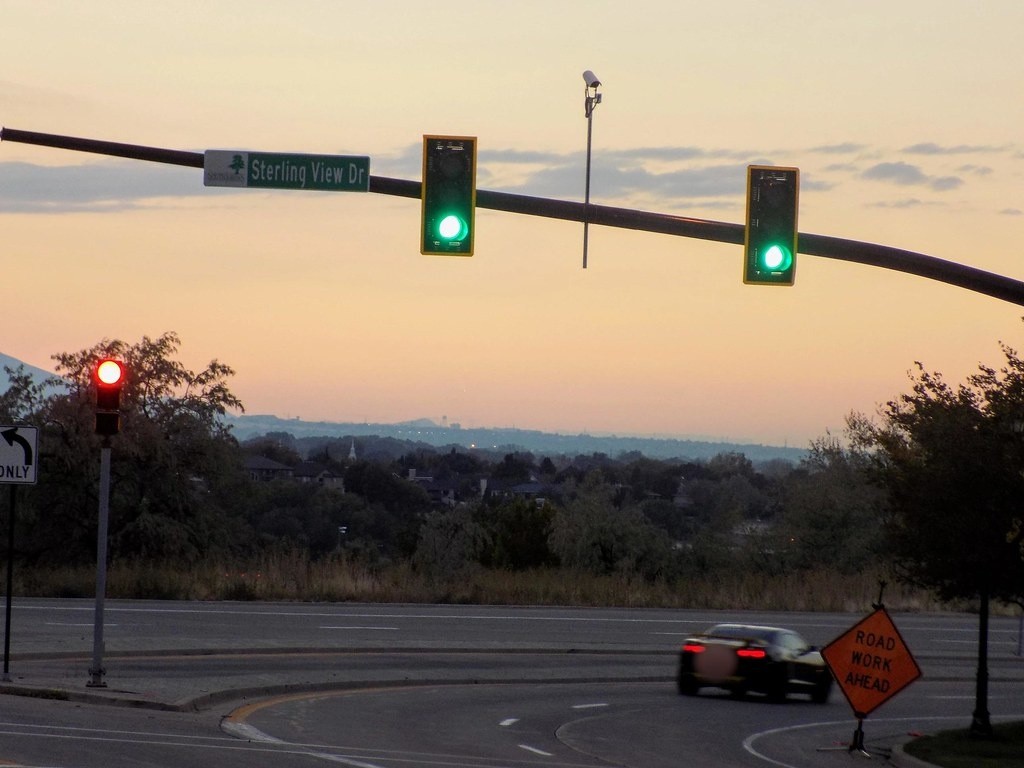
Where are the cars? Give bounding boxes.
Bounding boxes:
[677,624,833,710]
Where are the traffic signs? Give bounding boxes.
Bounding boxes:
[201,148,372,196]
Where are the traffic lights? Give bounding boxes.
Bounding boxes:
[94,358,128,440]
[419,134,478,258]
[742,162,802,287]
